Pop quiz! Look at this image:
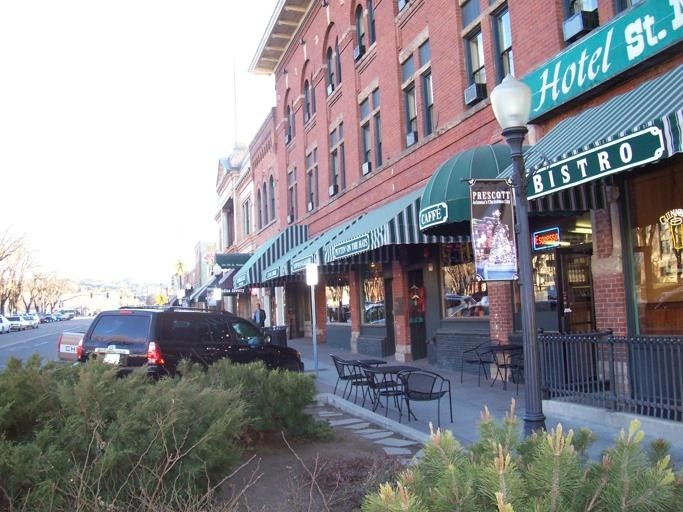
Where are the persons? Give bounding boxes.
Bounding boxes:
[253,303,266,328]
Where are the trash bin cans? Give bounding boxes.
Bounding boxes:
[260,326,288,347]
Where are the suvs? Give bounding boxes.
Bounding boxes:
[76,305,306,397]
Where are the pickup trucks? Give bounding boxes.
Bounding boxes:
[57,305,197,364]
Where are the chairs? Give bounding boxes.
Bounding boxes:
[460,339,525,396]
[330,352,454,430]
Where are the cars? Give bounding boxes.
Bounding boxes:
[328,290,488,330]
[0,308,80,337]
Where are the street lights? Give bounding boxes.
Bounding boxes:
[484,70,555,451]
